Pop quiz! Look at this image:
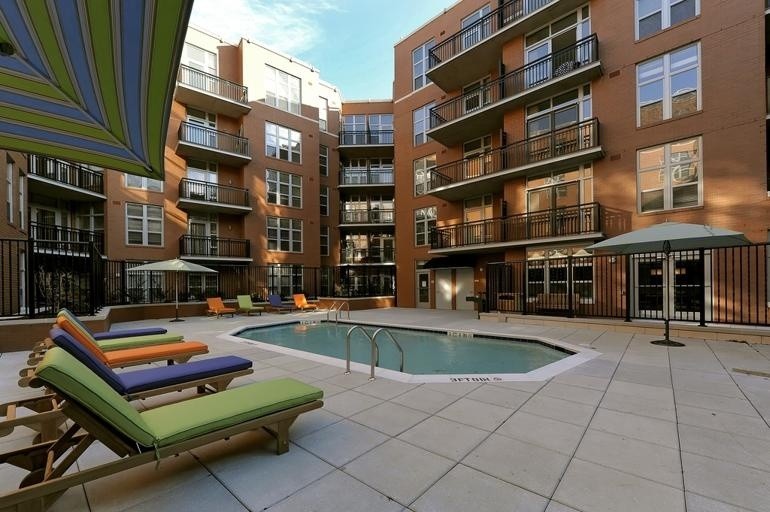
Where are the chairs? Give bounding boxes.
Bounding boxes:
[26,311,184,366]
[1,348,324,512]
[206,296,235,319]
[234,293,265,316]
[265,293,296,314]
[290,293,317,313]
[18,317,210,395]
[33,308,168,352]
[1,328,256,440]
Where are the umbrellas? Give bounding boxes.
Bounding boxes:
[0,0,194,182]
[583,220,753,346]
[124,257,219,322]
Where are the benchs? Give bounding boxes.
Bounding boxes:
[533,293,580,318]
